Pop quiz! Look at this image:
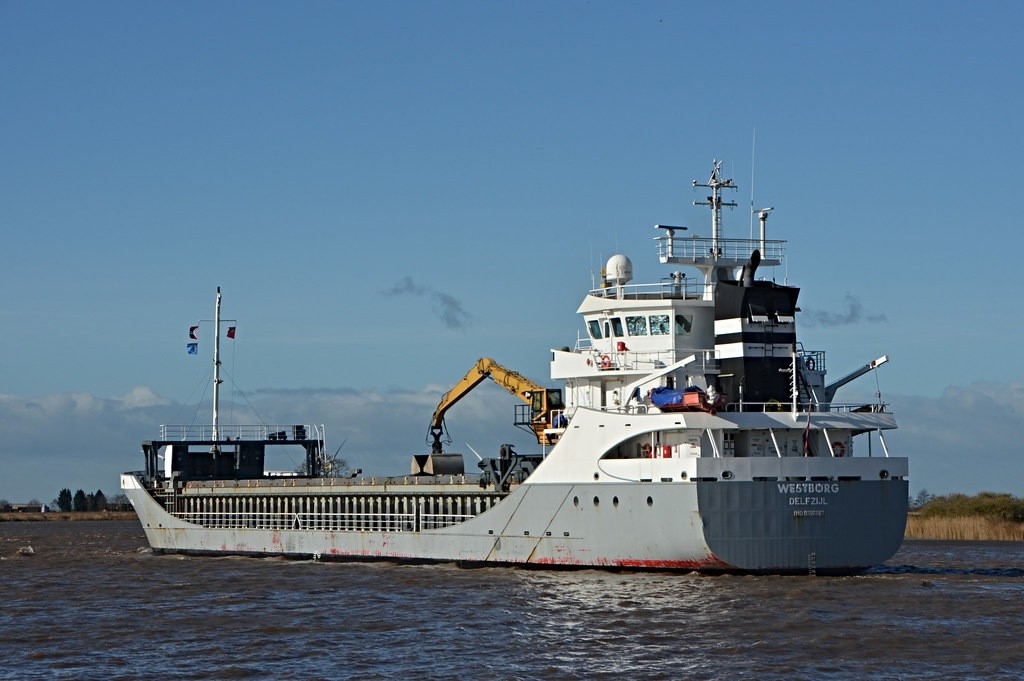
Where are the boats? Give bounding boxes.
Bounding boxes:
[118,128,909,570]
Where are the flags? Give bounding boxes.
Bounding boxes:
[186,343,197,354]
[189,326,199,339]
[227,327,235,339]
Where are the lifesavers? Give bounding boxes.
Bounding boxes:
[806,357,816,370]
[642,443,651,458]
[601,356,611,371]
[831,441,846,458]
